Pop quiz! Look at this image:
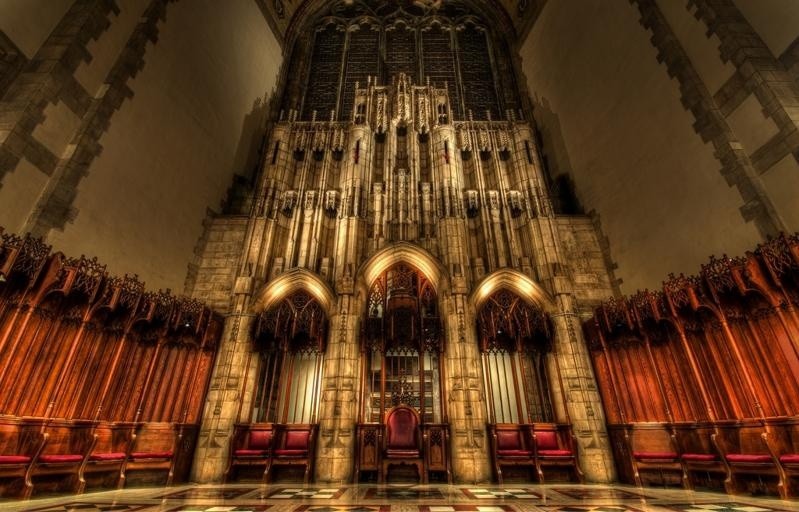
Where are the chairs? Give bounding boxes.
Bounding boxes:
[0,404,799,512]
